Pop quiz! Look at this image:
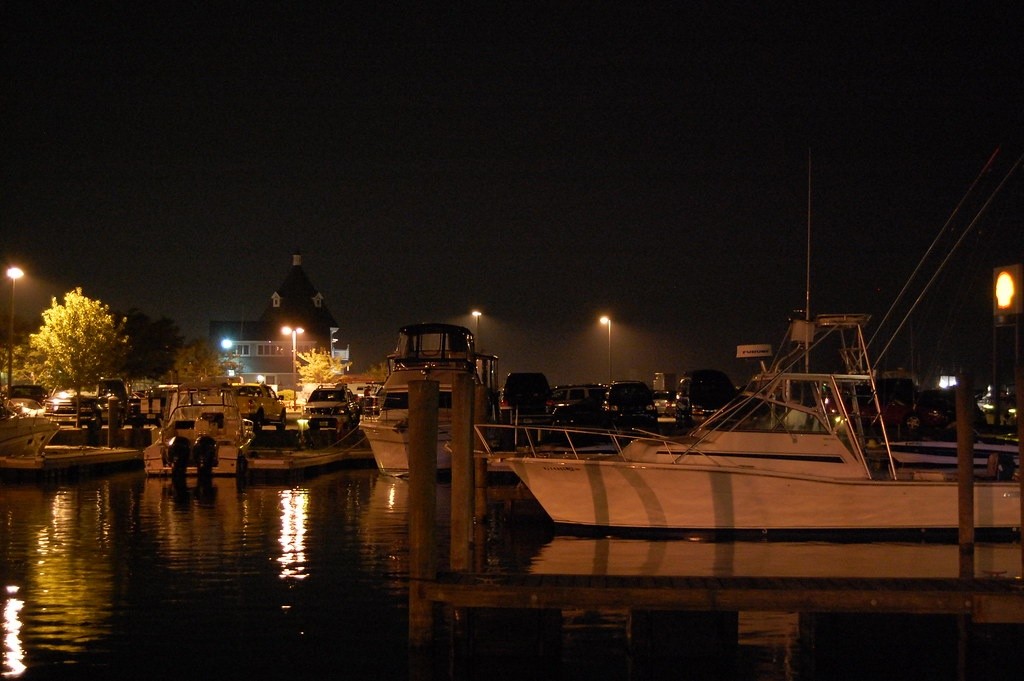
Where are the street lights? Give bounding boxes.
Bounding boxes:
[471,309,484,353]
[6,267,22,393]
[283,328,304,411]
[598,314,613,382]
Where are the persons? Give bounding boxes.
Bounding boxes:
[364,386,371,396]
[781,383,808,429]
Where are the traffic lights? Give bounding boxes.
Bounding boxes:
[990,263,1022,316]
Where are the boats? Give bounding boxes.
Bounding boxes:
[473,147,1023,535]
[522,525,1023,662]
[0,390,146,473]
[358,320,500,476]
[141,380,254,478]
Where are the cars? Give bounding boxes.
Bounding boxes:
[551,386,608,414]
[667,401,679,414]
[227,382,286,428]
[841,388,1020,439]
[4,375,152,419]
[654,389,672,416]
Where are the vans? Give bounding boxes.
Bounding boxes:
[502,371,556,426]
[676,369,740,427]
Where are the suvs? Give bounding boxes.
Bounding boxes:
[608,380,659,431]
[304,384,357,433]
